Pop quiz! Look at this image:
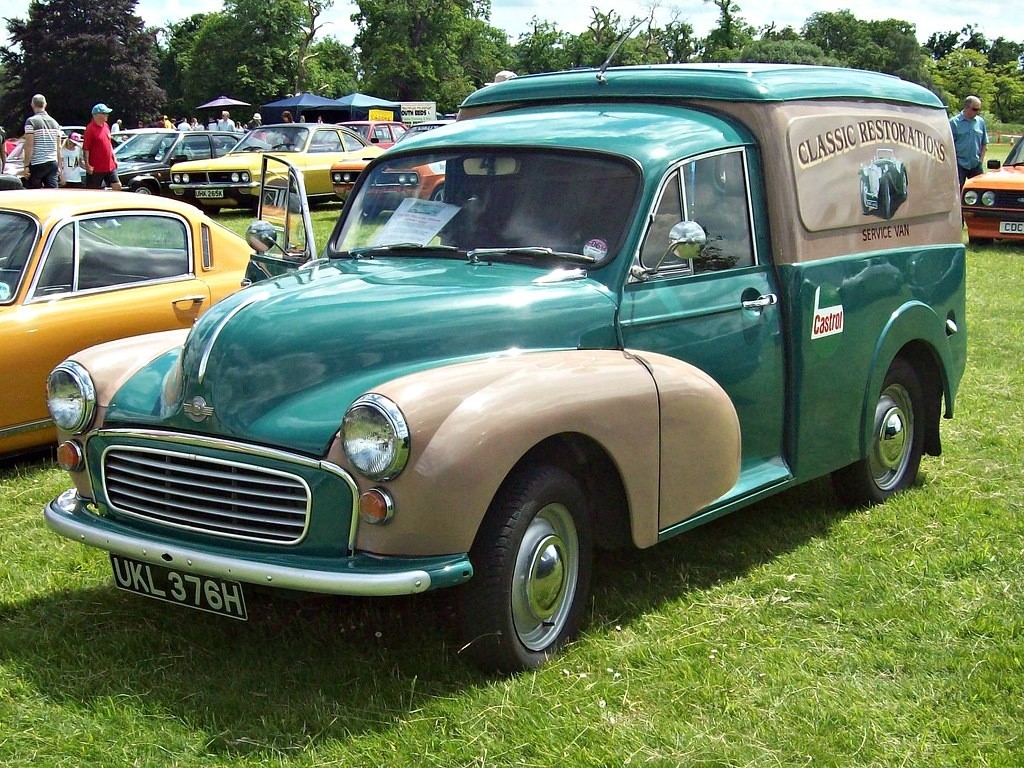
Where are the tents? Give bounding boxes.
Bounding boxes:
[335,91,401,122]
[259,93,352,124]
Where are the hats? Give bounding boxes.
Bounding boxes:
[70,131,82,147]
[91,103,113,115]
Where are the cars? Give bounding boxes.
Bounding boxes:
[42,58,970,678]
[961,133,1024,248]
[1,184,262,456]
[0,90,465,206]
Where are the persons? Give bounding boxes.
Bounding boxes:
[23,94,63,189]
[236,121,244,131]
[248,112,263,129]
[58,132,93,188]
[82,103,123,190]
[949,96,990,230]
[206,114,219,132]
[217,110,236,132]
[281,110,296,124]
[317,116,323,124]
[111,119,123,132]
[157,114,204,131]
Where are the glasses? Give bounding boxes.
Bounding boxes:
[282,115,286,117]
[220,115,224,116]
[968,105,981,112]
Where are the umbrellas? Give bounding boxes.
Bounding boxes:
[195,94,252,111]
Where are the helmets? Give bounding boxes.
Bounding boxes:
[253,113,262,119]
